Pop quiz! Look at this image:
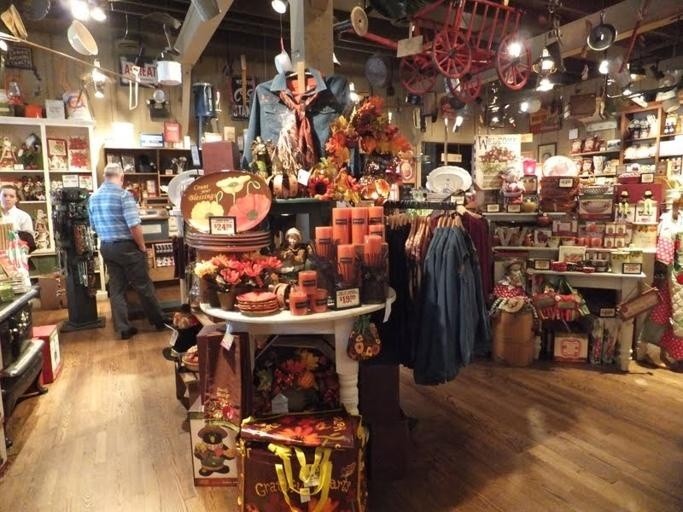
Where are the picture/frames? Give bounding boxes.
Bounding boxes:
[537,141,558,163]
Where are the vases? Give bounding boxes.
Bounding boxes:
[199,279,268,310]
[286,395,305,411]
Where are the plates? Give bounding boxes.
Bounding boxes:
[180,170,274,254]
[541,154,579,177]
[425,165,472,203]
[235,290,280,317]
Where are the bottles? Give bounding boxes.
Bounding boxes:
[7,82,25,117]
[633,120,675,139]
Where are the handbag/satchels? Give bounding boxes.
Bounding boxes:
[533,292,590,321]
[619,288,663,322]
[347,318,382,360]
[236,409,369,512]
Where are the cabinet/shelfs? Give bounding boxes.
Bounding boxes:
[482,211,657,346]
[0,116,243,315]
[570,95,683,179]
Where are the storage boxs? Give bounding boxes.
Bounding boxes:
[553,328,589,364]
[475,159,508,191]
[615,181,664,220]
[32,323,63,384]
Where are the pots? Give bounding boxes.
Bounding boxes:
[586,10,618,52]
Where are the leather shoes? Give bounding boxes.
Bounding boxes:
[121,327,138,339]
[156,312,174,330]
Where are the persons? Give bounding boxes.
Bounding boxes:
[0,184,38,246]
[88,164,176,338]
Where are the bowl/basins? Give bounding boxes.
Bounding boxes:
[67,19,99,56]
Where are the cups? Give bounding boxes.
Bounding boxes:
[156,57,183,87]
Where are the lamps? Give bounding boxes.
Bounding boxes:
[67,1,285,84]
[537,1,647,96]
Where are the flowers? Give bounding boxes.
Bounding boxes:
[256,349,342,409]
[304,93,414,206]
[194,251,284,293]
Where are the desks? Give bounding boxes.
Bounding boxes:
[525,267,647,373]
[0,286,48,448]
[198,282,397,445]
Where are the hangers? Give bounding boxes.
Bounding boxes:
[386,198,467,245]
[286,49,319,98]
